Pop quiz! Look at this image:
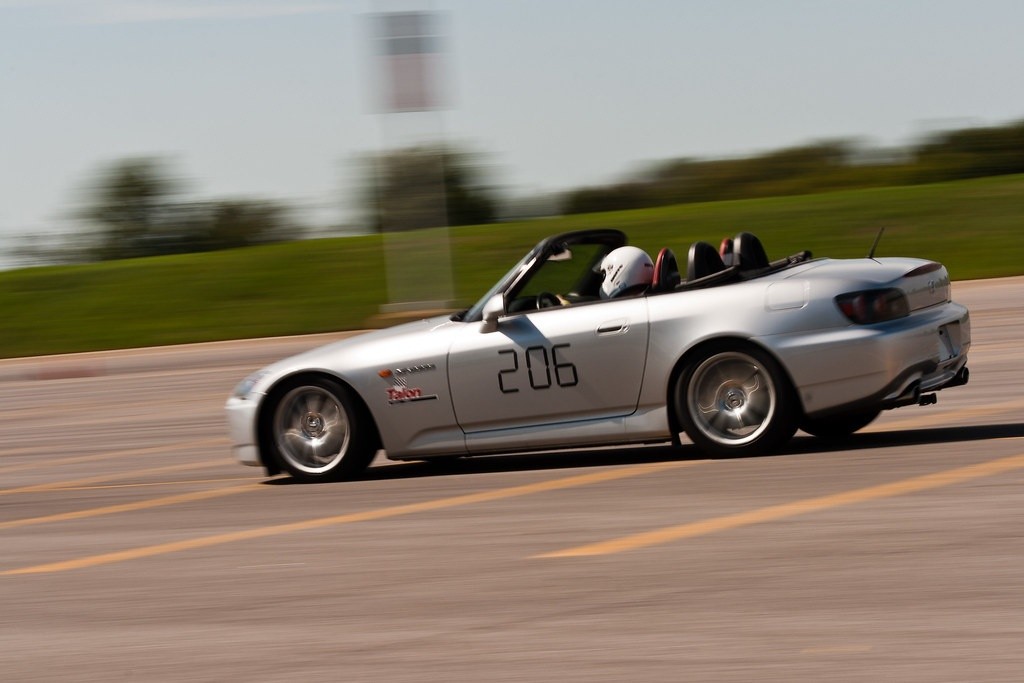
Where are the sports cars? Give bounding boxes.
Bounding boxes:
[226,230,972,483]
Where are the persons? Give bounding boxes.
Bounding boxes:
[555,246,654,307]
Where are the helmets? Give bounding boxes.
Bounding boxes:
[592,246,655,299]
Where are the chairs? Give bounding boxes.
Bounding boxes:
[687,240,727,281]
[732,231,770,272]
[644,247,681,298]
[717,237,735,270]
